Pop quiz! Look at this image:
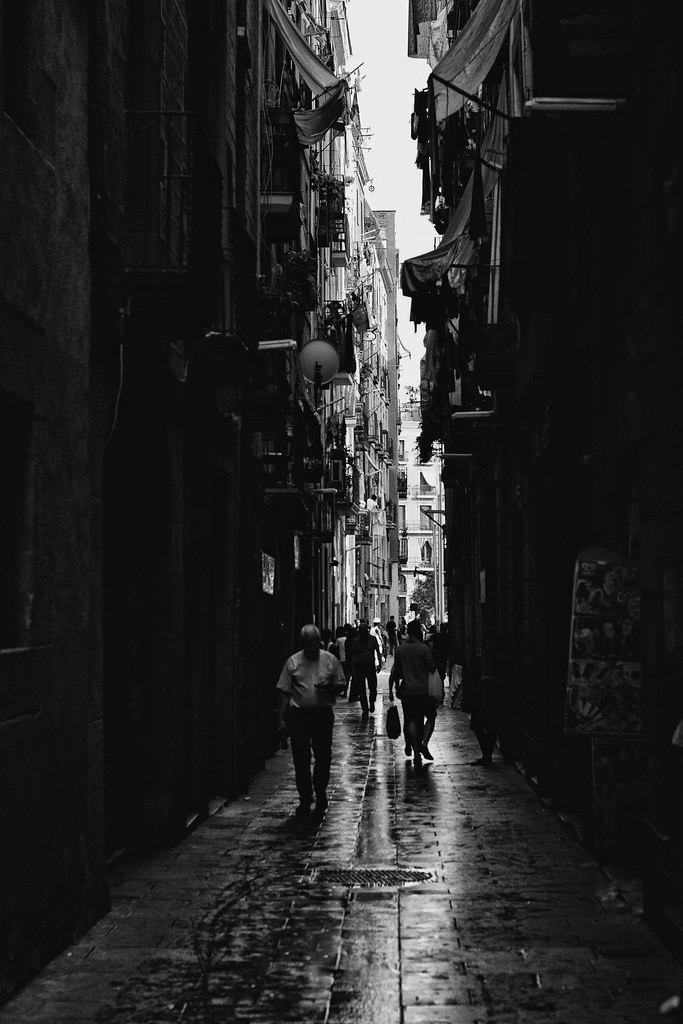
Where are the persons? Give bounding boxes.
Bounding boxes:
[322,616,450,704]
[395,620,437,763]
[463,631,510,764]
[389,631,424,756]
[275,624,347,815]
[352,625,382,717]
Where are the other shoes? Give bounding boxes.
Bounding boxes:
[420,746,433,760]
[362,711,368,715]
[296,799,311,813]
[470,757,493,766]
[483,743,494,761]
[405,749,411,756]
[414,758,422,763]
[370,702,375,713]
[314,788,327,808]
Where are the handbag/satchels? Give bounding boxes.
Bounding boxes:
[428,667,442,703]
[387,699,401,738]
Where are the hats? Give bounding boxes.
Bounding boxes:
[359,624,371,629]
